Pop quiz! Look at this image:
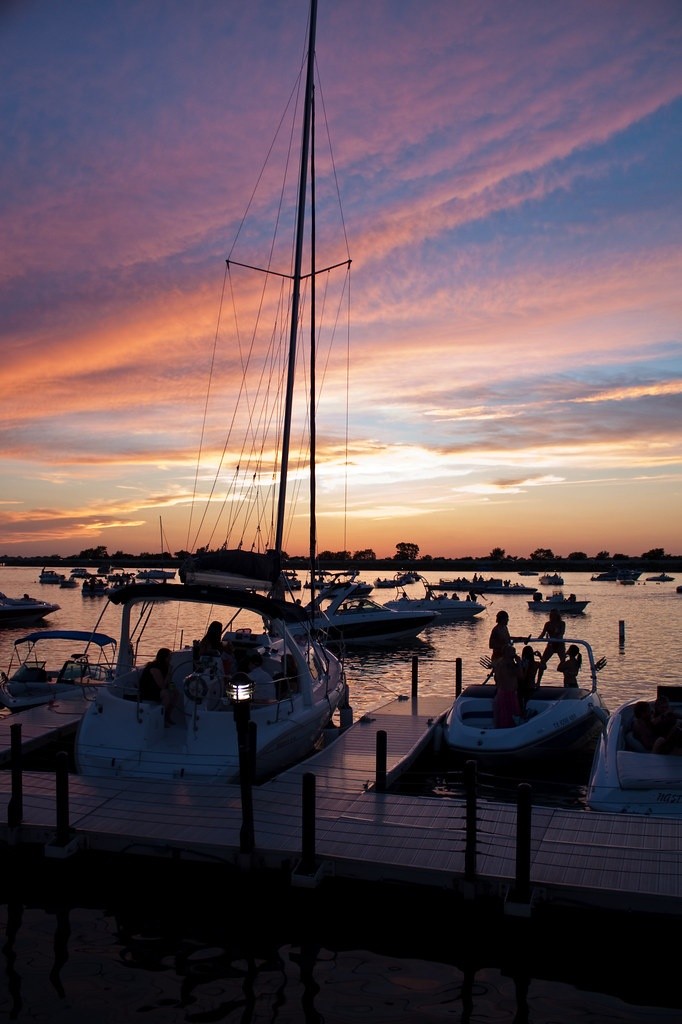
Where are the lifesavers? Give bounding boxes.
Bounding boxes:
[182,676,207,703]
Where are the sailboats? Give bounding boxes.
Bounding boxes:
[71,62,440,786]
[134,515,176,579]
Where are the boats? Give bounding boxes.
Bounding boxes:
[69,566,135,598]
[424,578,503,592]
[526,592,591,614]
[320,570,374,599]
[61,580,79,588]
[372,569,423,589]
[442,635,615,780]
[2,631,133,712]
[590,568,682,594]
[0,592,62,622]
[491,586,538,596]
[39,567,66,583]
[383,573,485,621]
[302,571,333,589]
[586,685,682,819]
[518,572,539,576]
[283,570,301,591]
[539,569,564,586]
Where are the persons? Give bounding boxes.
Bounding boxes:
[451,573,524,589]
[138,648,177,727]
[83,577,103,587]
[537,610,571,688]
[488,611,547,735]
[406,571,419,577]
[542,573,561,580]
[377,577,388,582]
[245,654,276,703]
[424,587,479,604]
[285,568,296,582]
[199,621,232,695]
[338,579,367,584]
[272,654,297,701]
[533,591,576,608]
[557,645,583,689]
[116,573,135,586]
[398,592,409,602]
[314,575,324,583]
